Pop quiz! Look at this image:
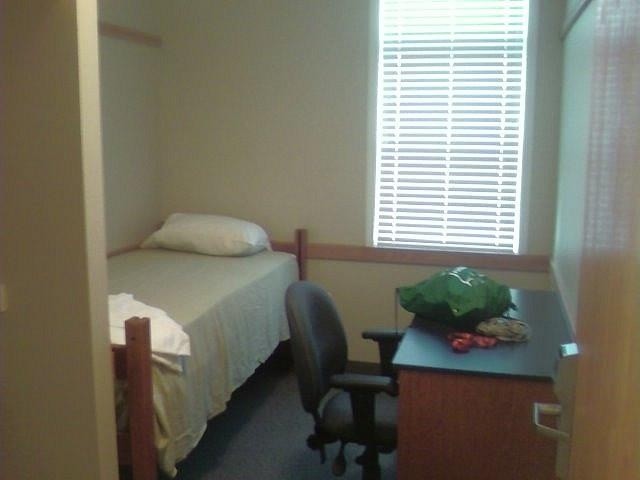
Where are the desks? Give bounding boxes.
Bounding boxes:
[392,287,573,479]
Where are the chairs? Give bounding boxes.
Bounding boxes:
[284,281,406,480]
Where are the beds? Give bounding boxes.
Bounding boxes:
[107,228,307,479]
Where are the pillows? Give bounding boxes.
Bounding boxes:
[139,212,272,256]
